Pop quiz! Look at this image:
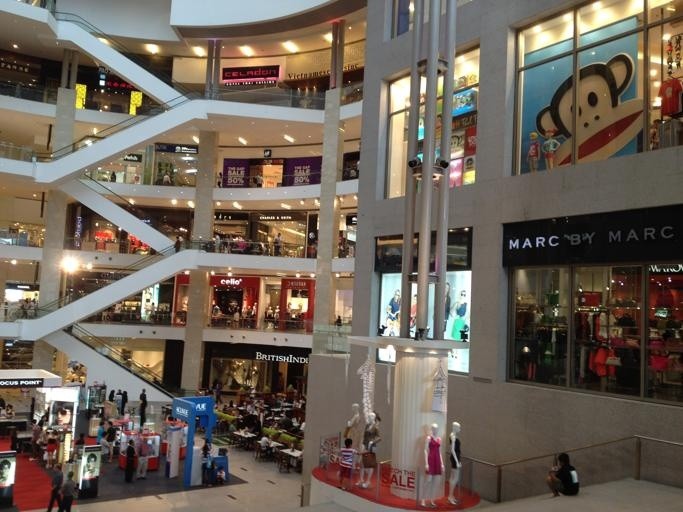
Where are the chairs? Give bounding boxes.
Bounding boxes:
[214,398,306,473]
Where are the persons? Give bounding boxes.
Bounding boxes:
[347,402,361,477]
[448,420,464,505]
[138,388,147,426]
[202,232,284,256]
[1,397,15,420]
[542,127,560,169]
[99,301,184,326]
[151,377,160,386]
[334,437,369,490]
[136,438,151,479]
[83,453,97,478]
[29,407,58,468]
[1,459,11,485]
[384,282,469,341]
[53,405,72,425]
[344,165,359,180]
[544,452,580,497]
[121,439,137,482]
[110,171,116,182]
[354,413,382,489]
[173,236,180,253]
[243,171,264,188]
[212,301,304,329]
[47,464,76,510]
[75,433,85,445]
[615,312,683,341]
[212,376,222,405]
[162,172,171,185]
[211,464,225,485]
[224,378,304,452]
[336,316,343,337]
[108,389,128,416]
[421,423,445,507]
[4,295,38,320]
[95,420,116,462]
[525,131,540,173]
[217,172,222,188]
